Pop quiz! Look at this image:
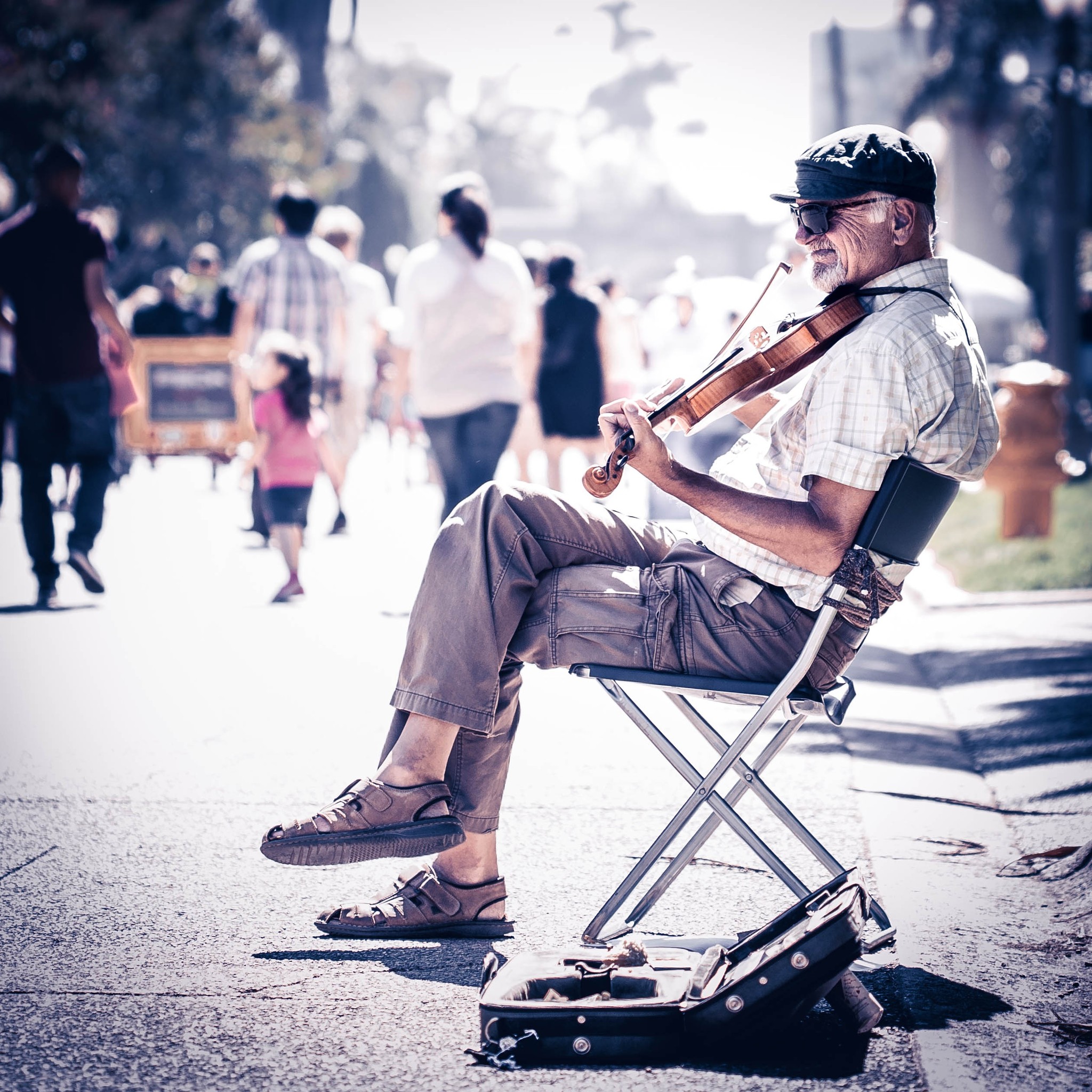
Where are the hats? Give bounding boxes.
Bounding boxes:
[769,124,936,206]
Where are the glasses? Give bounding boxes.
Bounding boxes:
[789,198,895,236]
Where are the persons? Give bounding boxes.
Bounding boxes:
[262,123,999,938]
[0,140,133,609]
[228,332,324,599]
[0,166,815,543]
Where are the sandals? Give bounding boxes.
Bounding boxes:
[260,777,467,866]
[315,861,517,939]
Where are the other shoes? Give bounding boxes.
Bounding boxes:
[68,549,105,592]
[272,573,304,602]
[38,585,57,608]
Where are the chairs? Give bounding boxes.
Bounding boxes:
[567,456,962,942]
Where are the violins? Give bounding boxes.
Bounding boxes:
[575,293,875,502]
